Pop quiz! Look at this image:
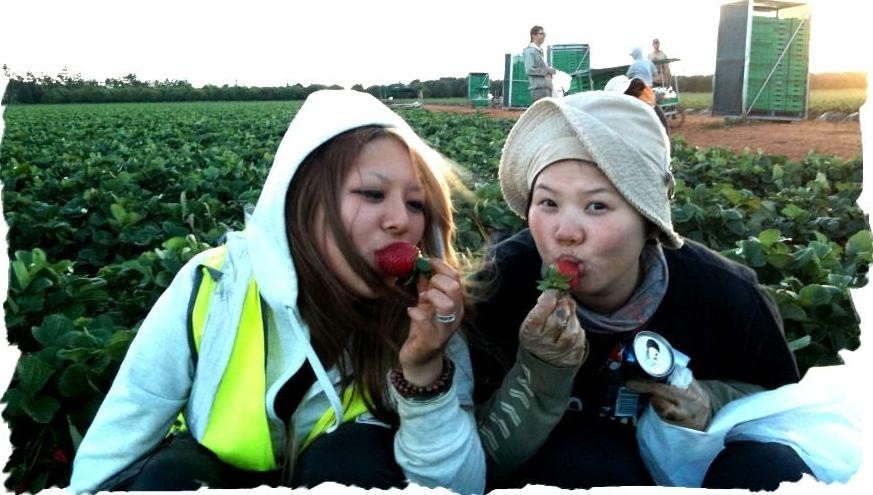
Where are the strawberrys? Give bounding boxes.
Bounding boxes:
[377,242,433,286]
[536,260,579,293]
[51,448,68,465]
[16,483,27,495]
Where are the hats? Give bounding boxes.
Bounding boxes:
[498,90,684,252]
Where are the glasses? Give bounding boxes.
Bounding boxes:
[537,33,542,35]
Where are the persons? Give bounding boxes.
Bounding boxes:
[521,25,556,102]
[647,38,671,88]
[64,88,492,495]
[459,88,812,495]
[625,47,659,88]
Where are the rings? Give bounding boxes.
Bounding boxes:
[435,312,456,324]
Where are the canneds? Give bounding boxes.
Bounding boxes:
[595,329,676,426]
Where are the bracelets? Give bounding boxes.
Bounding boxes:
[386,355,454,400]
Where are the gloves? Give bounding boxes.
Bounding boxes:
[623,375,711,433]
[517,287,587,368]
